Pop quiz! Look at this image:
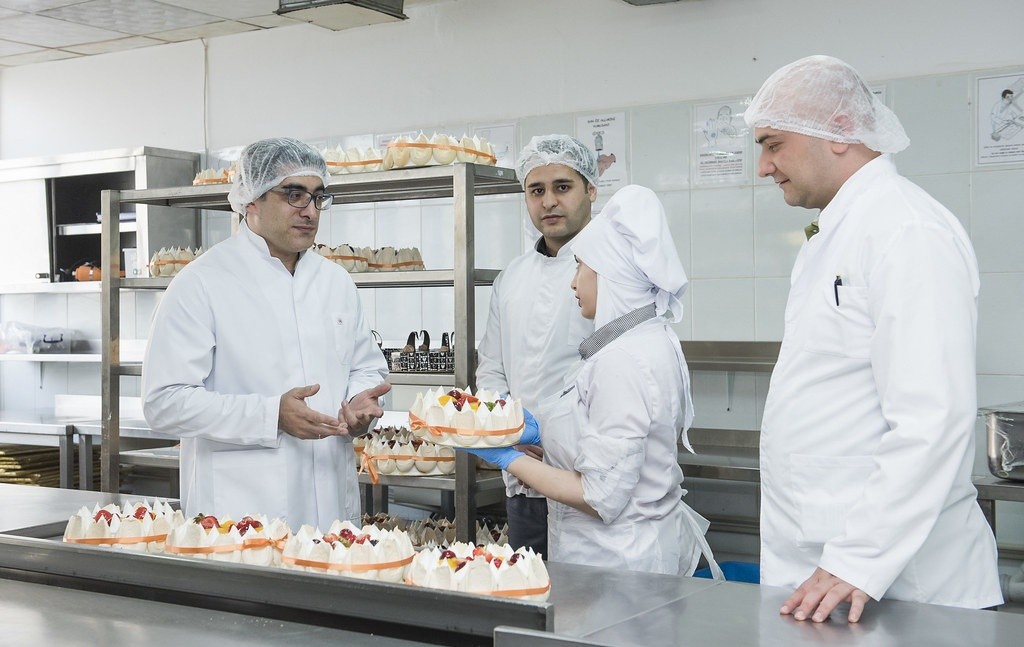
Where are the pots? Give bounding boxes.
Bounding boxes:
[978,407,1024,482]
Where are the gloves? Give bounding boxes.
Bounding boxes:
[454,446,525,470]
[518,408,541,444]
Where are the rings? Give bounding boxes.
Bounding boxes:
[317,433,321,439]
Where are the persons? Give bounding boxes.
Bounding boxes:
[743,57,1006,623]
[475,133,597,558]
[453,186,710,577]
[142,139,392,536]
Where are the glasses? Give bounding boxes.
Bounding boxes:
[269,189,334,210]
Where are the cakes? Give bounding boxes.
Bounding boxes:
[279,519,415,584]
[309,129,497,174]
[310,243,425,272]
[163,512,294,568]
[407,385,525,450]
[344,426,460,476]
[63,496,182,554]
[150,245,208,277]
[400,542,551,604]
[192,168,238,186]
[369,329,480,371]
[355,512,509,551]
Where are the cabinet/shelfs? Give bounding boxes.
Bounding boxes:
[0,146,201,491]
[99,163,526,544]
